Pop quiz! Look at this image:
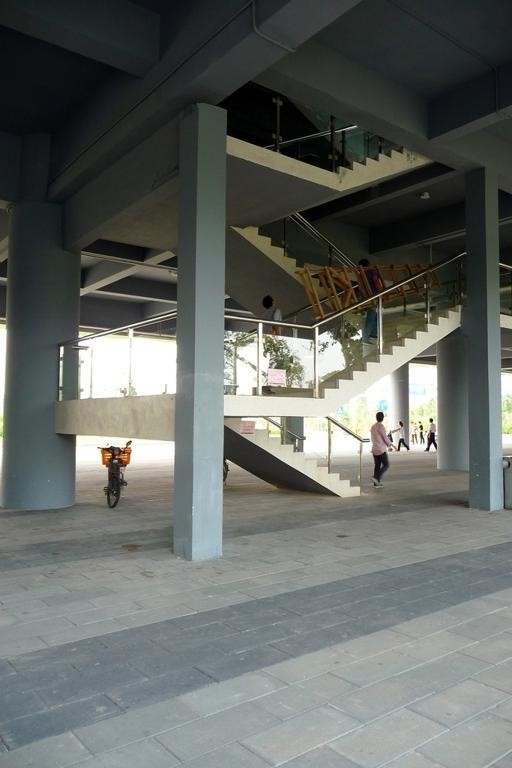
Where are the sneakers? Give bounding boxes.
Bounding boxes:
[368,477,385,489]
[396,442,430,452]
[262,385,276,395]
[360,336,379,346]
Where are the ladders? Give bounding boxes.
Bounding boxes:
[295,261,442,321]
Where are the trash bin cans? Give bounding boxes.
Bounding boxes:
[504,457,512,510]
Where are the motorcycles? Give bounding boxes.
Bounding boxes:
[97,439,135,509]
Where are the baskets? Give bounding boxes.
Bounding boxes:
[101,447,132,465]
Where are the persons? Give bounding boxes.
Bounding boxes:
[412,422,419,445]
[358,257,389,345]
[259,293,284,336]
[424,417,438,451]
[418,421,426,444]
[370,411,399,488]
[392,420,411,452]
